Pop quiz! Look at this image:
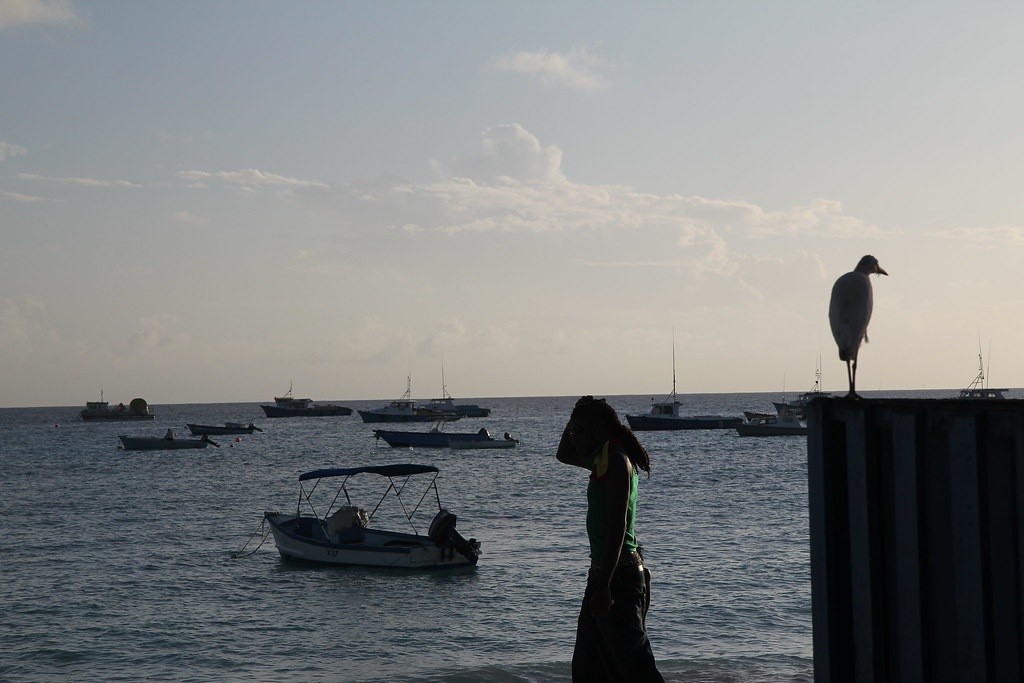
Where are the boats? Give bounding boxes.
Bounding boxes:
[625,327,832,437]
[118,423,254,451]
[260,380,353,418]
[80,390,157,422]
[264,464,482,571]
[946,329,1010,400]
[357,360,520,448]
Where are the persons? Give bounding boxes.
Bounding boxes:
[557,396,665,683]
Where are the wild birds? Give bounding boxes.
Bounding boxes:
[829,253,889,401]
